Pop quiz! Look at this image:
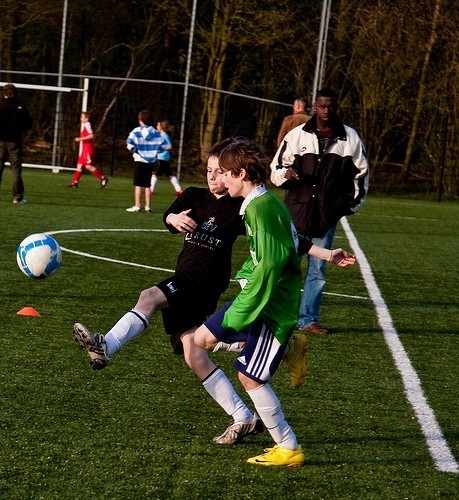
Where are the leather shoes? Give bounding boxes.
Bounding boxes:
[304,324,328,335]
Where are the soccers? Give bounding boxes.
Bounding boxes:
[17,234,63,279]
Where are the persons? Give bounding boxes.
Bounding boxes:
[268,88,371,338]
[276,94,314,152]
[74,136,356,446]
[0,85,33,204]
[149,118,184,197]
[193,141,310,466]
[125,109,168,213]
[68,112,109,191]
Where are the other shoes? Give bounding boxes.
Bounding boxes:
[145,207,151,212]
[67,183,78,188]
[174,189,184,196]
[101,179,108,188]
[126,206,141,212]
[13,197,27,204]
[150,191,155,195]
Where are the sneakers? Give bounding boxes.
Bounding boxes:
[73,323,112,370]
[247,444,305,468]
[213,413,263,444]
[285,334,307,386]
